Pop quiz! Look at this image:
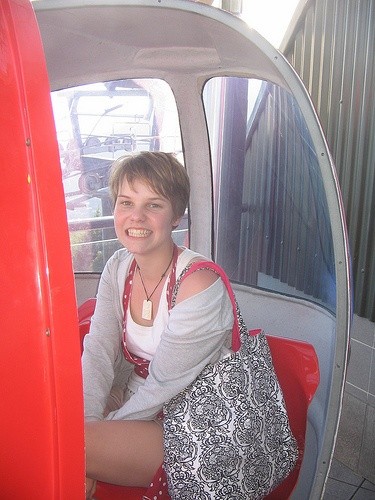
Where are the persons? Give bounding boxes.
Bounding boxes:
[79,151,250,500]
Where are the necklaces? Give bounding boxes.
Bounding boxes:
[134,242,176,320]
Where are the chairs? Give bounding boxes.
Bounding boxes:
[86,136,136,153]
[77,298,320,500]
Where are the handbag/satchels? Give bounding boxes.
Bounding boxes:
[164,260,299,500]
